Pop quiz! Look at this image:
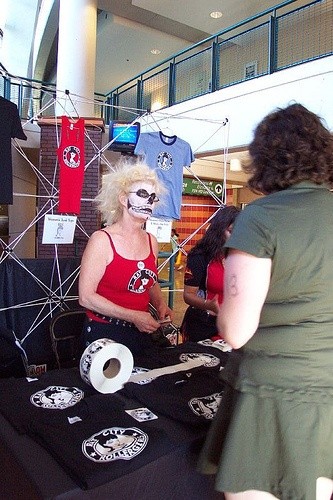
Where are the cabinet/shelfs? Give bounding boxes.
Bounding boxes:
[157,236,178,311]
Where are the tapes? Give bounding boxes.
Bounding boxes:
[81,338,135,394]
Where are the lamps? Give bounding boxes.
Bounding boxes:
[229,158,242,171]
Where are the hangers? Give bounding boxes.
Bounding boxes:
[50,96,102,133]
[159,114,176,138]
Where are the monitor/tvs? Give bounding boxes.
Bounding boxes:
[108,120,140,152]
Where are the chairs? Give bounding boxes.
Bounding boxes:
[49,308,88,369]
[0,326,27,378]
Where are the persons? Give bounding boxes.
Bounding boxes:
[180,205,241,342]
[216,102,333,500]
[78,159,175,359]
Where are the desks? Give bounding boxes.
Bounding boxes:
[0,335,240,500]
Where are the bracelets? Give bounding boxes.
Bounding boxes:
[204,300,206,304]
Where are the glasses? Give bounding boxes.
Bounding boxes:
[129,191,160,202]
